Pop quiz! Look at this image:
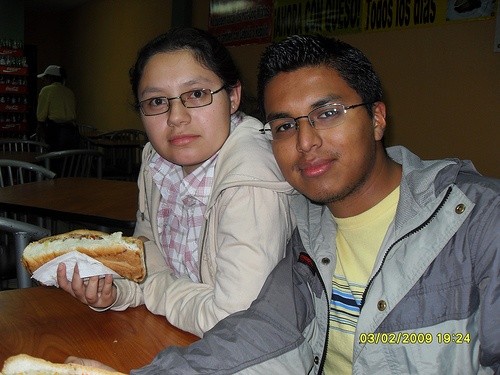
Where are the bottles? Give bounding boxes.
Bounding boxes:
[0,40,30,138]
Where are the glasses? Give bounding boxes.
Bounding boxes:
[136,83,228,117]
[258,101,377,142]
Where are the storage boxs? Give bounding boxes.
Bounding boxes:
[0,48,23,56]
[0,65,29,77]
[0,83,28,94]
[0,122,27,130]
[0,102,28,113]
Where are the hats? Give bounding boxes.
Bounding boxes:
[36,64,61,78]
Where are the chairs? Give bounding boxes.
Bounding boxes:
[0,122,148,289]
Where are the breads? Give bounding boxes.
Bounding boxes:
[0,353,130,375]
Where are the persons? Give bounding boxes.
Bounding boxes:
[62,34,499,375]
[36,65,81,176]
[56,29,296,338]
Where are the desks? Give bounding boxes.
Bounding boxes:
[0,151,44,163]
[0,177,140,229]
[87,138,145,179]
[0,285,203,375]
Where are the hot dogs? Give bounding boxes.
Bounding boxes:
[21,230,147,283]
